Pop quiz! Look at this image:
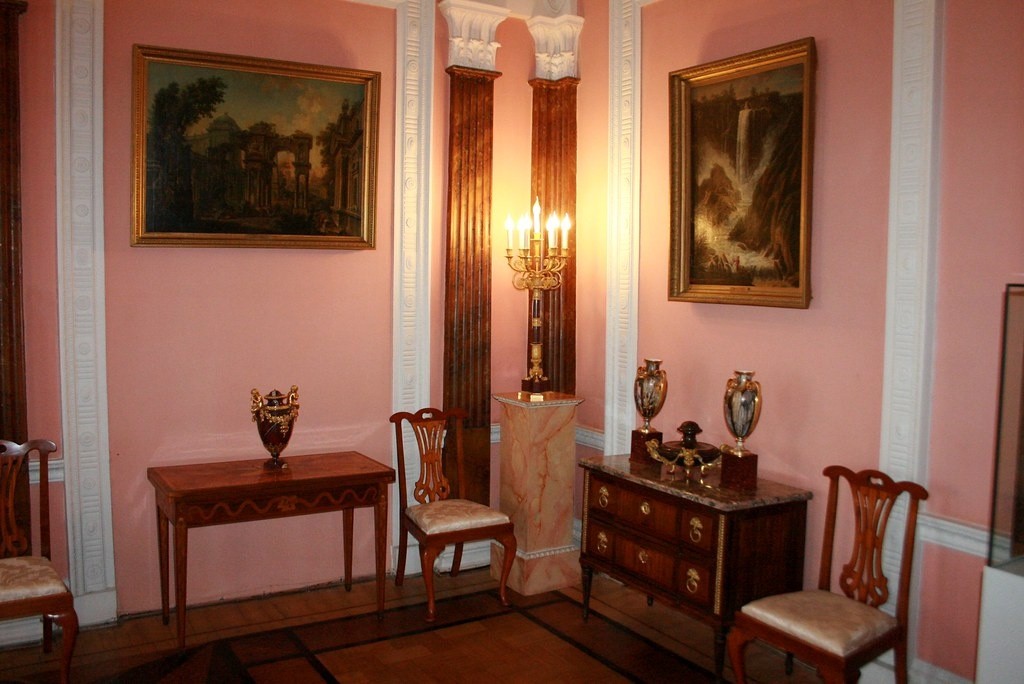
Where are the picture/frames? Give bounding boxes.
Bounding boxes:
[131,42,382,251]
[666,37,817,310]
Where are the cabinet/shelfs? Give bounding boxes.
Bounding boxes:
[577,453,814,684]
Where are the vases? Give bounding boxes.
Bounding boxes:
[723,369,763,458]
[633,357,667,434]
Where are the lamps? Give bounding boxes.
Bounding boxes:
[503,196,572,392]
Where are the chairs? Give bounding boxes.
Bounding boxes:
[0,439,79,684]
[390,408,517,622]
[726,464,928,684]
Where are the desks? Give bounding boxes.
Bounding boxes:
[493,392,584,597]
[147,450,397,651]
